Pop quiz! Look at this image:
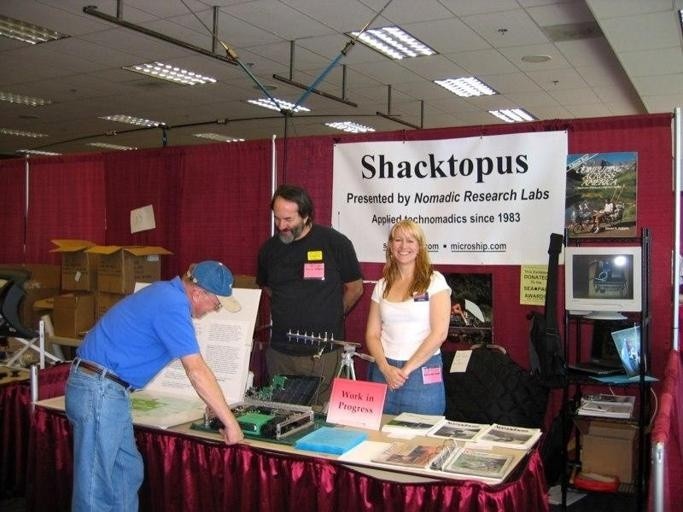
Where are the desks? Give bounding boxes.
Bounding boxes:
[35,396,547,511]
[1,359,71,511]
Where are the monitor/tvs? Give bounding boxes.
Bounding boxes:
[565,247,642,320]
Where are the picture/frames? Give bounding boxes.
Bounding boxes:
[565,152,638,237]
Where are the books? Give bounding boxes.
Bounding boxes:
[295,426,367,455]
[578,394,635,418]
[371,412,542,483]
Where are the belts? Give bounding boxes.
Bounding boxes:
[71,358,135,392]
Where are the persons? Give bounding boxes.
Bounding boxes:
[591,198,614,234]
[365,219,452,415]
[256,185,364,404]
[66,260,244,512]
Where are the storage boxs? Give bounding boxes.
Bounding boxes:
[232,274,262,327]
[0,239,174,338]
[579,418,641,484]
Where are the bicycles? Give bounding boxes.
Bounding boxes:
[570,207,598,233]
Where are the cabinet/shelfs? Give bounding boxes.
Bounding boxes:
[561,229,653,511]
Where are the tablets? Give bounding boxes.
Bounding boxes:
[271,374,324,405]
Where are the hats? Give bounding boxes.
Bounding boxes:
[186,259,242,314]
